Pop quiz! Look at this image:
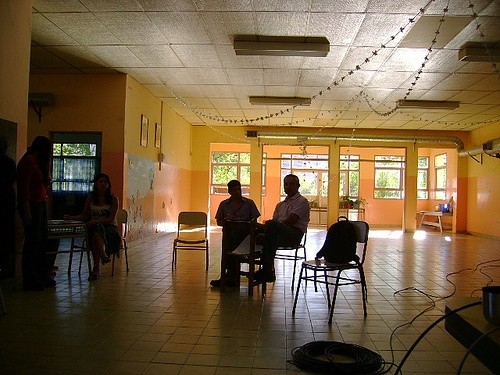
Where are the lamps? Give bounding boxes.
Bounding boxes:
[397,42,500,110]
[234,34,331,57]
[249,96,311,106]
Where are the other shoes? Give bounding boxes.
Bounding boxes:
[30,273,56,290]
[211,275,240,288]
[246,268,275,282]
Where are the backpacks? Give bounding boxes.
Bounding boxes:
[315,215,356,264]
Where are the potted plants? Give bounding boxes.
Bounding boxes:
[340,197,368,209]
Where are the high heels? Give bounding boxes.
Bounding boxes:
[101,256,111,265]
[88,271,99,281]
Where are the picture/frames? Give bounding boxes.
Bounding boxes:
[140,114,161,148]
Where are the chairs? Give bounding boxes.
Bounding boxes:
[221,218,368,325]
[172,212,208,271]
[79,209,129,277]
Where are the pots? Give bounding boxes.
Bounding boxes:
[481,286,500,327]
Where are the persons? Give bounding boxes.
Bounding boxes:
[221,175,311,286]
[64,173,119,281]
[210,180,261,287]
[17,136,56,292]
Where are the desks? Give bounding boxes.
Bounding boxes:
[310,207,365,227]
[47,220,92,274]
[419,212,451,234]
